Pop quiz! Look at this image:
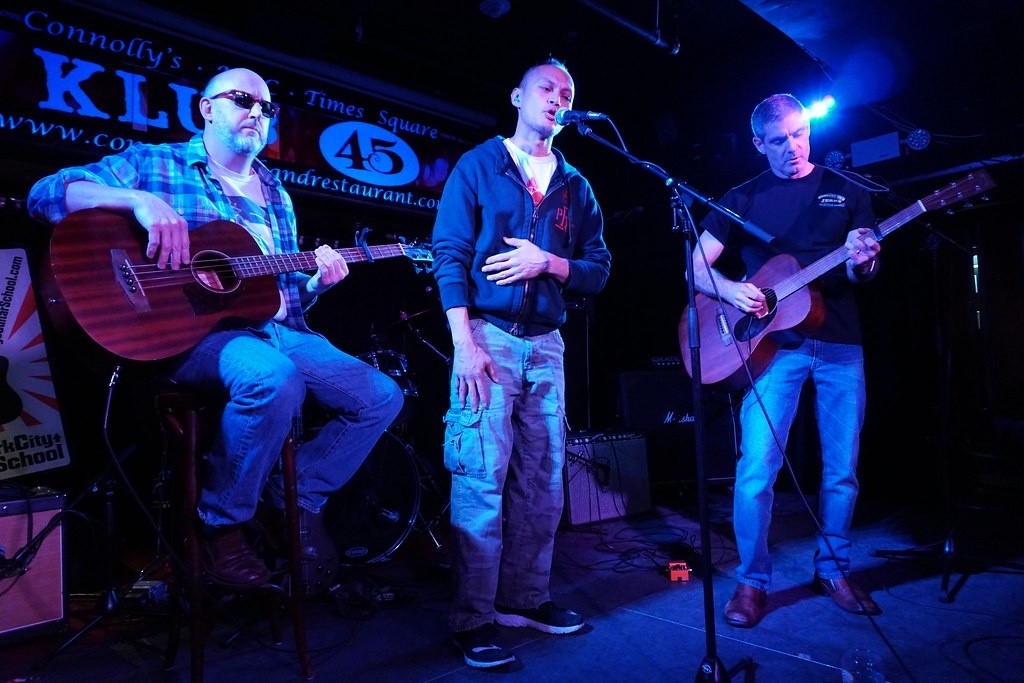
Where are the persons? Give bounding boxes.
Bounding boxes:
[27,66,404,582]
[682,94,882,629]
[432,57,613,667]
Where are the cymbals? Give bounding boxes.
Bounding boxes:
[382,308,431,337]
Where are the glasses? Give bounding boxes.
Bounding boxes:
[210,89,280,118]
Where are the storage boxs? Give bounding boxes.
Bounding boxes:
[0,482,69,646]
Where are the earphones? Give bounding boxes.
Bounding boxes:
[515,97,520,102]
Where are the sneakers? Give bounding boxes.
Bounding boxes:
[451,623,516,667]
[495,603,585,634]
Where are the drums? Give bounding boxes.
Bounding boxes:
[306,424,422,567]
[352,348,421,425]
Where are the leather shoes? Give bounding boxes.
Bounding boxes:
[813,571,876,613]
[199,526,269,587]
[283,506,341,574]
[723,582,767,626]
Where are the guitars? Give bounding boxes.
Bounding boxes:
[36,208,436,381]
[676,166,1001,395]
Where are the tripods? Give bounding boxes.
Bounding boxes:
[0,442,213,671]
[867,193,1024,604]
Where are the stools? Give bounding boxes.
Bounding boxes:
[156,383,319,683]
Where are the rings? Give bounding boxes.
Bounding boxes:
[855,250,862,254]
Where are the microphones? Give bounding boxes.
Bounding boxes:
[367,495,401,525]
[602,461,610,493]
[555,108,609,125]
[0,544,32,580]
[369,326,382,351]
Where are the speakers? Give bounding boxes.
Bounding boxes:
[618,365,742,492]
[561,430,652,528]
[0,485,69,643]
